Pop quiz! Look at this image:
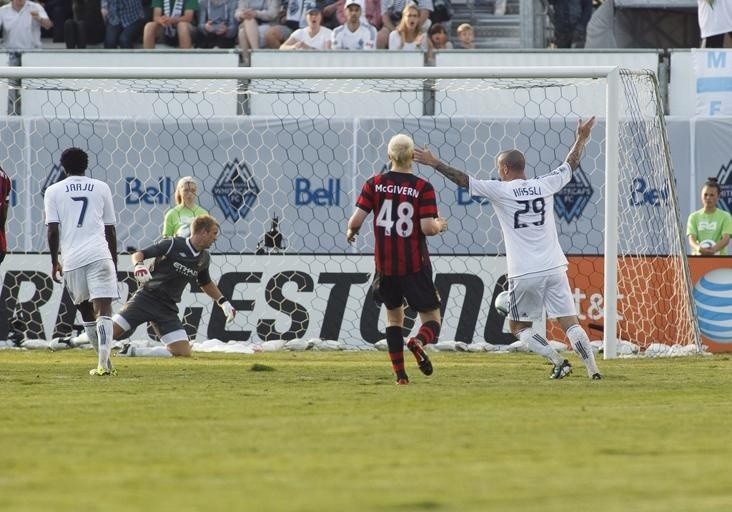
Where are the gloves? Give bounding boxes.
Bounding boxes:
[134,262,153,283]
[216,295,237,324]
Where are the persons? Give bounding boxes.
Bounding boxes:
[543,0,602,49]
[686,176,732,255]
[345,134,450,387]
[411,115,604,380]
[49,214,237,357]
[161,174,214,240]
[695,0,732,49]
[0,165,11,267]
[44,146,120,378]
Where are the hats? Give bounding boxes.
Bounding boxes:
[304,1,323,15]
[344,0,364,11]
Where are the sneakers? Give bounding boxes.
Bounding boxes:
[406,337,433,376]
[590,372,604,380]
[90,367,119,377]
[116,342,133,357]
[549,359,573,380]
[396,377,409,385]
[48,336,80,351]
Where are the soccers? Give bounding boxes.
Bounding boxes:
[698,239,720,255]
[494,291,511,316]
